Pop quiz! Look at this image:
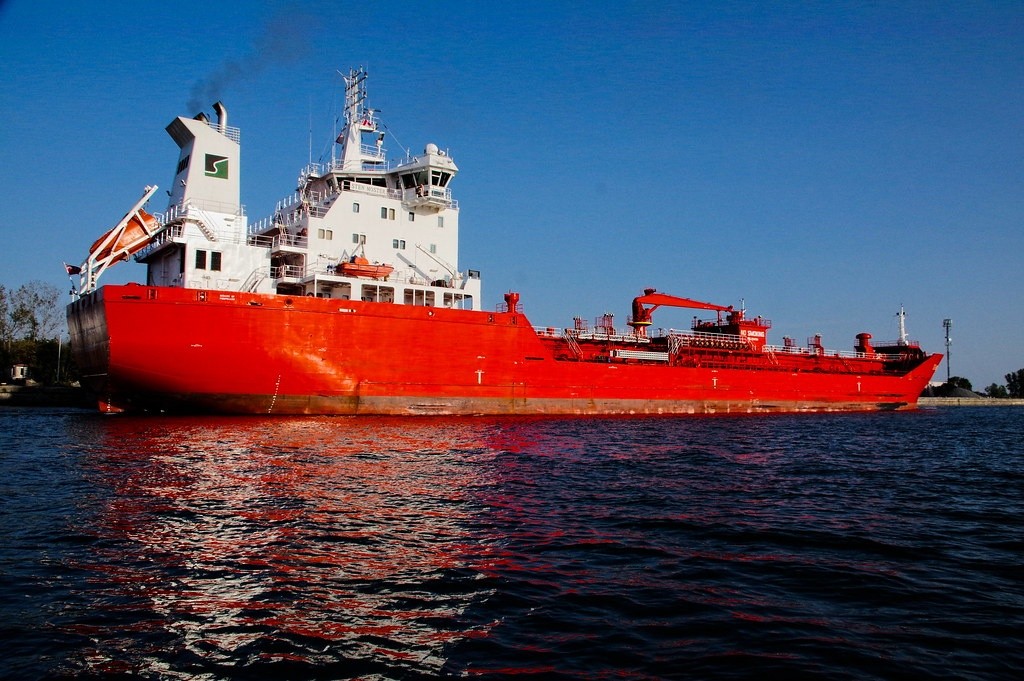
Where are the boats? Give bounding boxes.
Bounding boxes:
[62,66,944,423]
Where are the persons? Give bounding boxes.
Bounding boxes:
[417,182,425,196]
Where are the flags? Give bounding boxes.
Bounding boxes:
[362,119,375,128]
